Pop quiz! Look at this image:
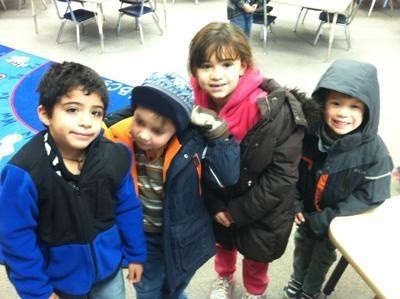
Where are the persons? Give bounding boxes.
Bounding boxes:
[105,70,242,299]
[227,0,257,38]
[187,21,320,299]
[0,60,145,299]
[286,57,392,298]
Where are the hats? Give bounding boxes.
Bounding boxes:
[132,73,195,132]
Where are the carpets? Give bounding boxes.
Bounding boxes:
[0,45,134,176]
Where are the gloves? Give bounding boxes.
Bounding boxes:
[191,104,229,140]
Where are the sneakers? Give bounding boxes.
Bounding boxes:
[210,276,236,299]
[283,276,302,299]
[298,290,320,299]
[242,290,267,299]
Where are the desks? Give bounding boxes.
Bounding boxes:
[322,195,399,298]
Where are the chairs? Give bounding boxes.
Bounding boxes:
[246,0,277,46]
[116,0,164,45]
[57,0,98,51]
[294,0,362,58]
